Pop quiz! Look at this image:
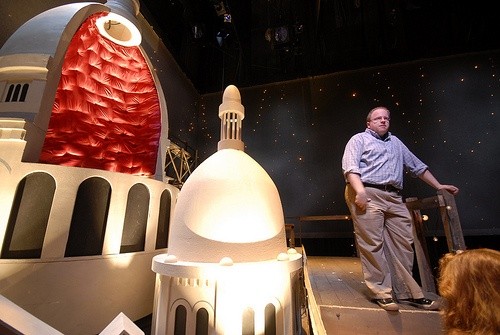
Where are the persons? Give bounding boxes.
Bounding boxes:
[437,248,500,335]
[341,107,459,311]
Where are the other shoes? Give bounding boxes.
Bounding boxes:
[398,297,435,307]
[376,298,396,307]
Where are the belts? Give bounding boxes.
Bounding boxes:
[362,182,401,193]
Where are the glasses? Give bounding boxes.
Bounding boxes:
[368,116,390,122]
